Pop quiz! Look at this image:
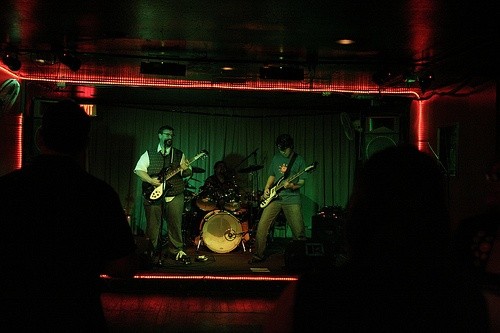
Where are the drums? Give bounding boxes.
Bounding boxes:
[219,192,241,212]
[199,210,242,254]
[196,190,217,211]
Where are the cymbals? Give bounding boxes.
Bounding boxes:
[237,165,264,174]
[192,166,205,173]
[185,184,196,189]
[192,177,201,183]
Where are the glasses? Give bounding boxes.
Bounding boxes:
[161,133,174,136]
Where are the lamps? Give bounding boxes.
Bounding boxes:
[60,49,81,71]
[3,51,21,71]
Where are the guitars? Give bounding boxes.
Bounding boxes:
[258,161,318,209]
[143,149,207,203]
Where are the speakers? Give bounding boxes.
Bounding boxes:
[30,98,63,157]
[356,114,401,179]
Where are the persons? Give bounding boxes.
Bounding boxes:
[133,125,193,263]
[0,100,140,333]
[252,134,307,262]
[204,161,236,190]
[291,142,491,333]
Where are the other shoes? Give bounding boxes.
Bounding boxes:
[248,255,265,264]
[174,252,189,263]
